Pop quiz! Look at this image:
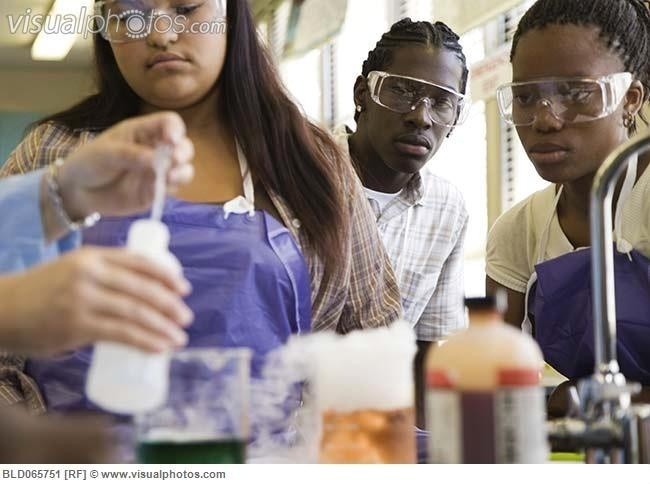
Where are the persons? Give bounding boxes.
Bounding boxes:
[486,1,649,331]
[1,2,401,438]
[0,109,198,355]
[331,17,470,430]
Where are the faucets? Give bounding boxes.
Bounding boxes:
[573,136,649,464]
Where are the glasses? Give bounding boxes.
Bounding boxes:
[366,69,472,127]
[494,71,636,126]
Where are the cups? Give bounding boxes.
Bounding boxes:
[136,335,417,464]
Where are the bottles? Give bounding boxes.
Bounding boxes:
[423,294,543,463]
[82,219,183,416]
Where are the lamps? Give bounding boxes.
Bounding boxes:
[29,1,88,63]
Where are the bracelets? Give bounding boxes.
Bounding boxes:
[42,158,101,232]
